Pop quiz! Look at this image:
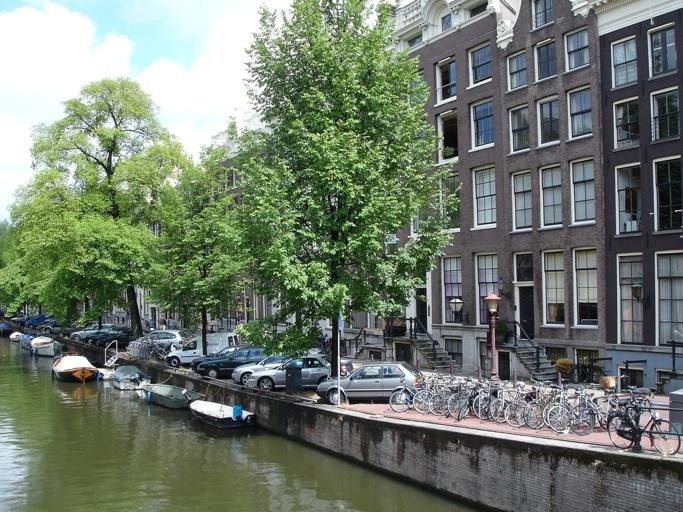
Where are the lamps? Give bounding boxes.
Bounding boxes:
[499,276,503,295]
[449,299,468,323]
[632,282,649,308]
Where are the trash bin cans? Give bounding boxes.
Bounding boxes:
[287,365,302,395]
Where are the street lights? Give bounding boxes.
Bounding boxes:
[482,292,501,383]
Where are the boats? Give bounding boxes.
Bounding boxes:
[142,382,204,408]
[52,353,103,385]
[110,365,151,391]
[0,321,64,358]
[188,399,254,431]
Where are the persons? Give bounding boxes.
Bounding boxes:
[340,365,347,376]
[159,314,167,330]
[146,318,156,330]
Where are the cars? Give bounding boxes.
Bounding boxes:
[313,360,425,406]
[3,311,152,350]
[126,328,352,394]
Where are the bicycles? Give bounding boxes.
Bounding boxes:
[386,368,681,458]
